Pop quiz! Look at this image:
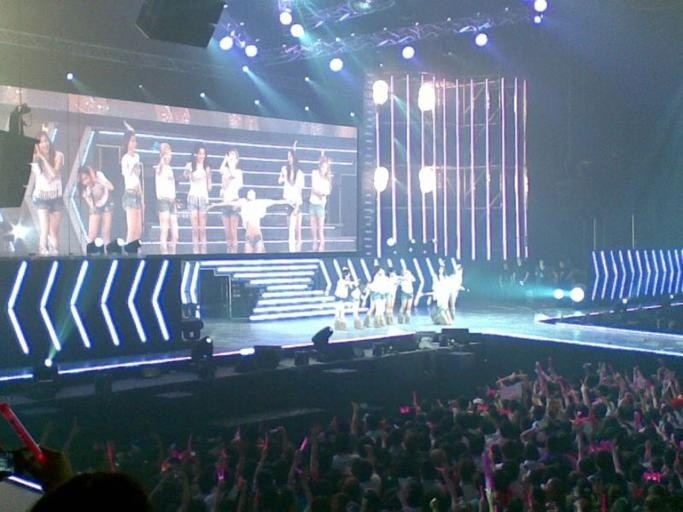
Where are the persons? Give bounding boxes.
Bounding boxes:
[31,130,65,254]
[183,142,212,254]
[308,157,332,251]
[1,311,683,510]
[217,149,244,254]
[278,149,306,252]
[334,259,466,330]
[77,166,115,255]
[209,188,291,253]
[154,142,180,255]
[497,258,572,306]
[120,131,145,256]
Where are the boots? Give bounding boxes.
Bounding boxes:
[354,315,411,330]
[335,319,348,331]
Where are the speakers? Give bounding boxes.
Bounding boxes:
[1,130,41,208]
[442,328,469,345]
[134,0,225,47]
[255,345,281,361]
